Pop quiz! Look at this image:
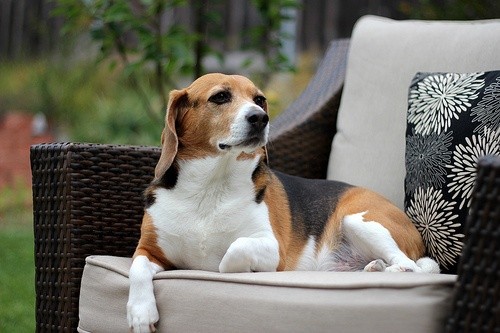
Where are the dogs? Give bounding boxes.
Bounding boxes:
[126,72,441,333]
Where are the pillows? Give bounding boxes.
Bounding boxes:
[403,71,500,276]
[326,15,500,210]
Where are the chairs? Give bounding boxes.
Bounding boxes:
[29,38,500,333]
